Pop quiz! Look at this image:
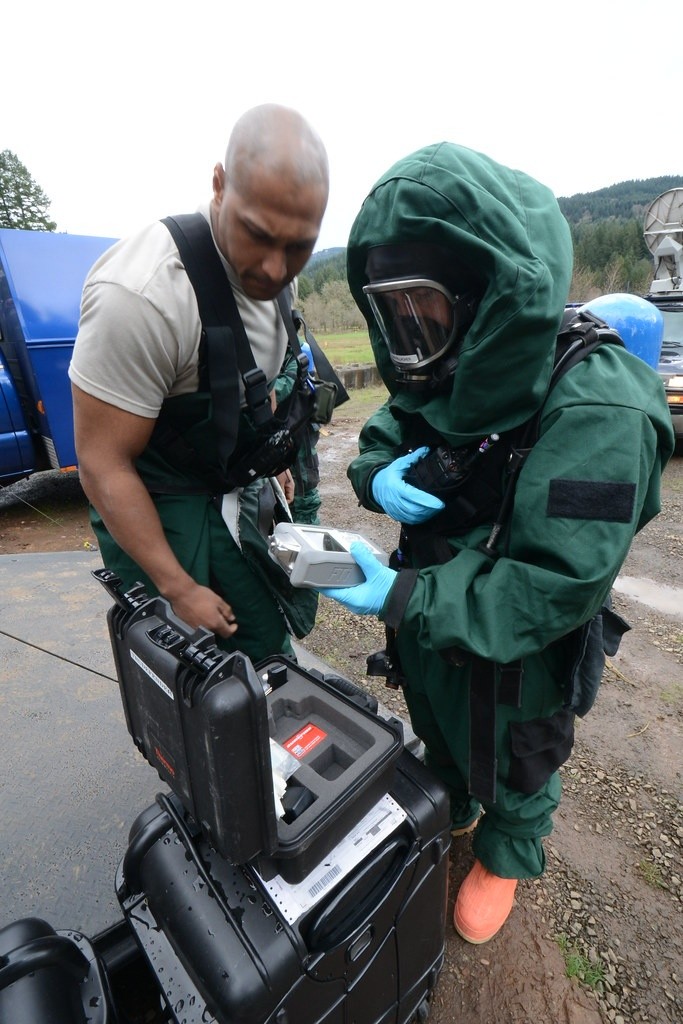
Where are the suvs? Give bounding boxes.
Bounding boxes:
[563,296,683,436]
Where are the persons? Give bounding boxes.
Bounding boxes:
[315,143,674,946]
[68,102,329,669]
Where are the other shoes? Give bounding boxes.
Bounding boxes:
[454,860,519,945]
[450,818,479,837]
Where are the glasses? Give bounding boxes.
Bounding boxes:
[362,272,486,381]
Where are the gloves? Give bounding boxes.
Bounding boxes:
[312,541,399,616]
[371,446,445,524]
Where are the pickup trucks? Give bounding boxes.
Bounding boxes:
[0,228,315,488]
